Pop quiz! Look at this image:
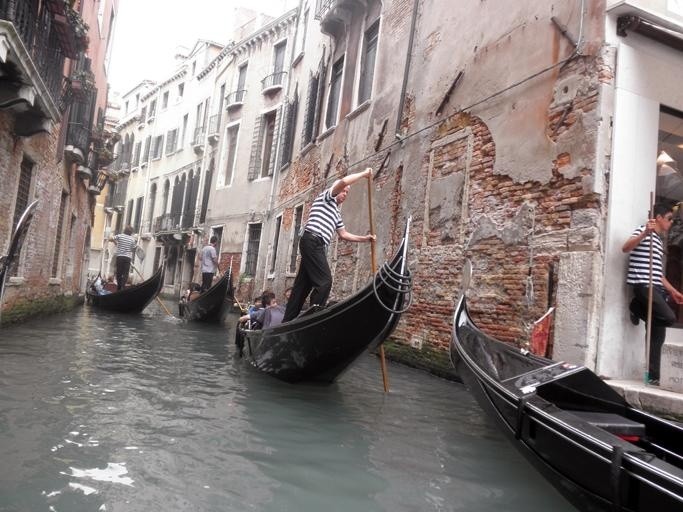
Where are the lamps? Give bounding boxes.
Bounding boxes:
[657,112,676,164]
[658,163,676,177]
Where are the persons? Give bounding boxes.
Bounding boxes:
[238,287,293,330]
[199,236,222,294]
[109,226,136,291]
[621,203,683,386]
[281,167,377,325]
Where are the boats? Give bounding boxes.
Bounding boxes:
[234,215,412,386]
[450,292,683,511]
[0,198,40,309]
[86,255,166,315]
[178,256,235,327]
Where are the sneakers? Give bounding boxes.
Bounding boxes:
[630,296,639,325]
